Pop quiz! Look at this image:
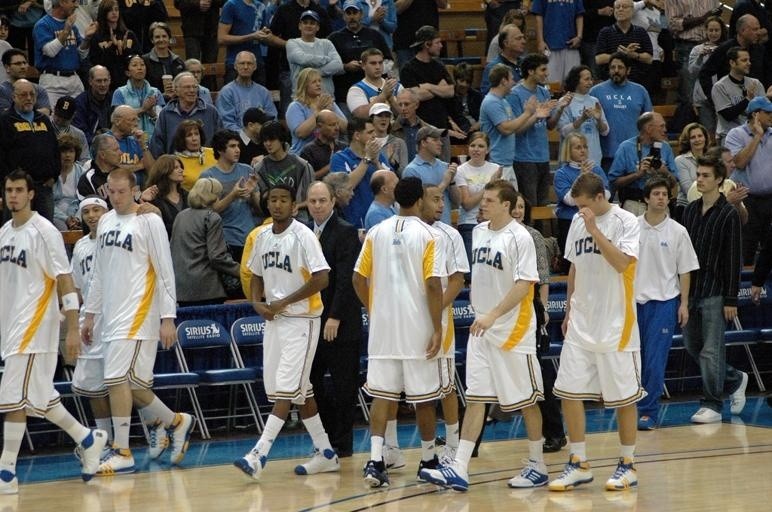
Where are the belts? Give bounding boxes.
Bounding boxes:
[39,68,76,78]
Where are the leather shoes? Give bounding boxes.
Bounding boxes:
[542,435,568,452]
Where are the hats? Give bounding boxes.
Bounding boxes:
[747,96,772,115]
[409,26,440,49]
[55,96,76,120]
[416,125,448,143]
[343,0,364,12]
[368,103,393,119]
[299,11,320,23]
[243,107,276,125]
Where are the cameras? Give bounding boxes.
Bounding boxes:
[647,148,662,170]
[703,46,714,54]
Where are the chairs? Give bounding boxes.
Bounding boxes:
[451,282,771,424]
[2,0,734,251]
[0,295,369,449]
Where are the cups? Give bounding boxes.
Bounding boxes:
[162,75,174,93]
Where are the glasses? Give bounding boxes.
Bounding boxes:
[8,60,29,66]
[126,53,143,64]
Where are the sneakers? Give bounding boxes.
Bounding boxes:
[507,458,551,488]
[294,447,339,476]
[637,413,656,429]
[691,407,723,424]
[549,454,595,491]
[234,447,268,481]
[604,456,639,491]
[364,436,477,492]
[0,469,20,495]
[729,370,749,415]
[75,412,197,485]
